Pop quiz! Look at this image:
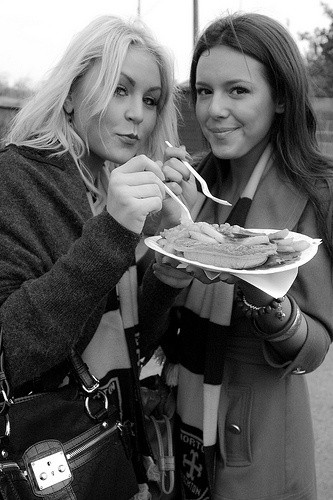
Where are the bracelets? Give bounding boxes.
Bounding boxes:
[243,293,266,311]
[247,295,303,344]
[235,280,283,314]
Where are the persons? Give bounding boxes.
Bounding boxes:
[0,17,197,499]
[138,13,332,500]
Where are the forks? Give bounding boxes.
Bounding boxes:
[165,139,233,207]
[155,173,195,227]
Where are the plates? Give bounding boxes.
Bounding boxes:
[143,226,319,275]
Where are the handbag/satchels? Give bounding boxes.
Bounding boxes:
[0,379,138,499]
